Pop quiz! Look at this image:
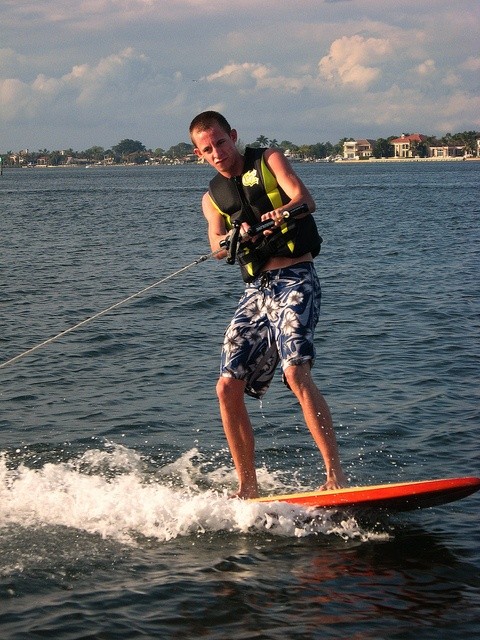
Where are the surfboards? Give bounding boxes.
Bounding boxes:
[244,476,479,508]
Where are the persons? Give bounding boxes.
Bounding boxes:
[188,111,351,501]
